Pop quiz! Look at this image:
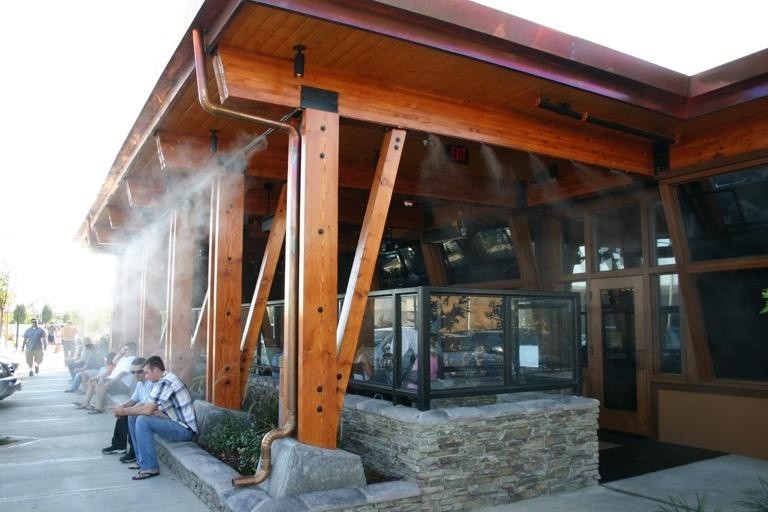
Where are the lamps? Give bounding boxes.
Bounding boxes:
[209,129,219,152]
[293,44,306,78]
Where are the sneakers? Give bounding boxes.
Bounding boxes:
[119,451,137,463]
[28,366,39,377]
[102,444,127,454]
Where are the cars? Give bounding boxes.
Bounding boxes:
[351,328,539,388]
[657,324,680,365]
[0,355,22,401]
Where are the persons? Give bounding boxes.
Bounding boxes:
[47,318,198,480]
[353,327,492,391]
[21,319,46,376]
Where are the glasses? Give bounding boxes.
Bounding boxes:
[130,370,144,375]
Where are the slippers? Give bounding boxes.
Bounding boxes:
[132,470,160,480]
[71,398,106,415]
[129,463,141,470]
